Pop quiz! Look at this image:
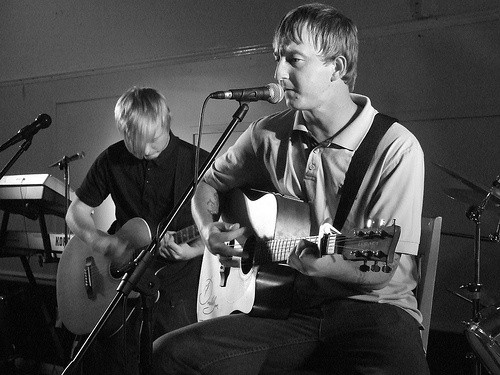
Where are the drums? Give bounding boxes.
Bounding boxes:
[463,305,500,375]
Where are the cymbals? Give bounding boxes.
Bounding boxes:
[432,161,500,201]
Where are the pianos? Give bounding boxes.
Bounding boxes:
[0,173,95,366]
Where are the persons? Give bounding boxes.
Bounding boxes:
[65,86,218,375]
[151,2,430,375]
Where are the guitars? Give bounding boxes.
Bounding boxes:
[195,186,402,319]
[55,216,199,336]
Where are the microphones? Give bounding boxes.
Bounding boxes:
[210,82,284,104]
[51,151,86,167]
[0,113,52,152]
